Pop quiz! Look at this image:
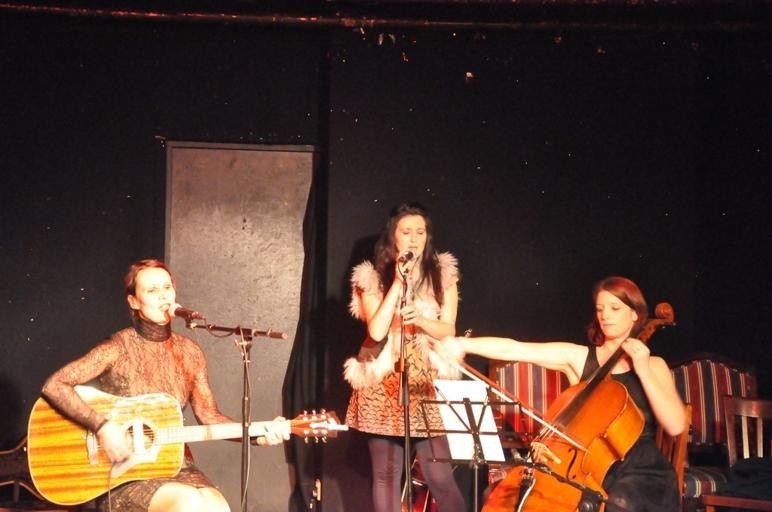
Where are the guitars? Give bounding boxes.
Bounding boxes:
[26,384,349,506]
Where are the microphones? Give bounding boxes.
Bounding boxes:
[398,250,416,263]
[168,303,204,320]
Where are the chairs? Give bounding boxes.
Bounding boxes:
[669,353,772,512]
[489,361,571,450]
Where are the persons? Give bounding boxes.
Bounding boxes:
[436,276,687,511]
[39,257,290,512]
[342,201,466,511]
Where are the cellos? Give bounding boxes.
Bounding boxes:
[480,303,676,512]
